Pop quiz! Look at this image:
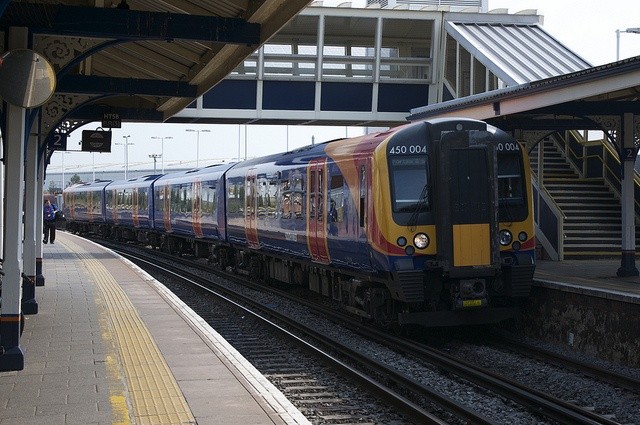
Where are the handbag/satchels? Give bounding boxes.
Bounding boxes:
[55,210,66,226]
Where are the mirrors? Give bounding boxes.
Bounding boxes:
[1,47,57,109]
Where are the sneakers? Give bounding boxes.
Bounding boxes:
[43,239,47,244]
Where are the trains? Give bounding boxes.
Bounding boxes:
[64,118,536,335]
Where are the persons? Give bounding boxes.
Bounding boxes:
[43,199,59,244]
